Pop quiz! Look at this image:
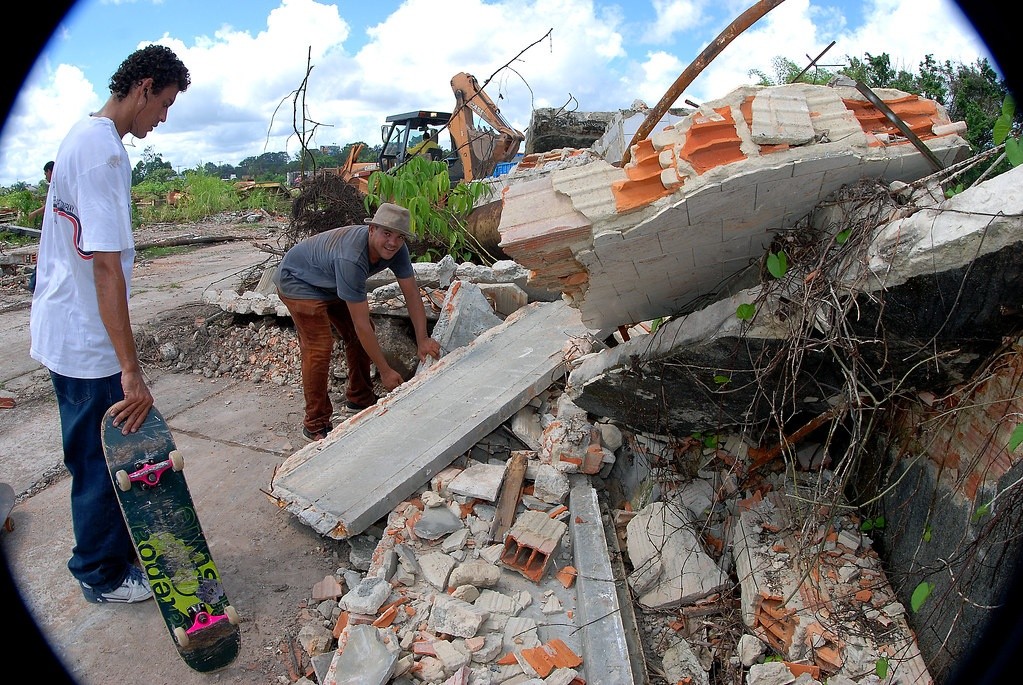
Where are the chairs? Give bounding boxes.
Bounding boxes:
[424,147,443,162]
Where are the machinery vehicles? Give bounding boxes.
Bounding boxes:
[334,72,525,205]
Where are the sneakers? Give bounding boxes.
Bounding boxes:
[79,562,154,603]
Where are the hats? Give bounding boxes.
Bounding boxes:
[364,202,416,237]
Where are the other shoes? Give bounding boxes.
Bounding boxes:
[302,423,330,443]
[346,393,381,414]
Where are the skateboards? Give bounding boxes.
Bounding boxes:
[100,398,241,674]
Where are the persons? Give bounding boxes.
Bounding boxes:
[406,133,439,155]
[274,202,441,443]
[20,161,54,294]
[29,45,192,603]
